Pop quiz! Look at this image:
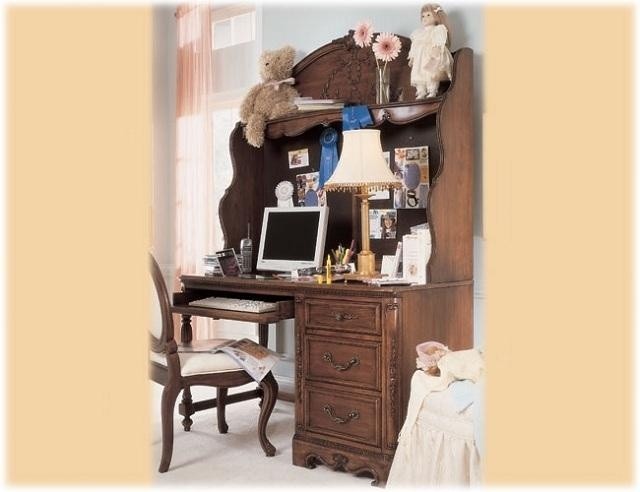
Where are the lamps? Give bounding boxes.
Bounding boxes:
[322,129,402,281]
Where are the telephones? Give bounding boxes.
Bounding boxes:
[235,223,256,279]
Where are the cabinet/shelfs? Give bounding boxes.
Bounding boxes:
[170,29,474,488]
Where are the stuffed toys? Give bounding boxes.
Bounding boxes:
[239,45,300,148]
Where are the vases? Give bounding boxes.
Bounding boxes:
[376,67,390,104]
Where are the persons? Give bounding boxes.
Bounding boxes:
[381,212,397,239]
[291,155,299,165]
[407,3,455,100]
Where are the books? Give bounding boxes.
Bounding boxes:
[178,338,279,383]
[202,247,241,277]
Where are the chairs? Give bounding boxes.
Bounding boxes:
[148,255,279,475]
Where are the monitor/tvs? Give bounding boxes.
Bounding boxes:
[255,207,329,278]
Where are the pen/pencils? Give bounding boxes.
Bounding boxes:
[330,239,355,265]
[327,254,332,285]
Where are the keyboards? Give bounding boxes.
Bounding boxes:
[189,297,277,314]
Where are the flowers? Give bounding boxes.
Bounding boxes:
[352,18,401,101]
